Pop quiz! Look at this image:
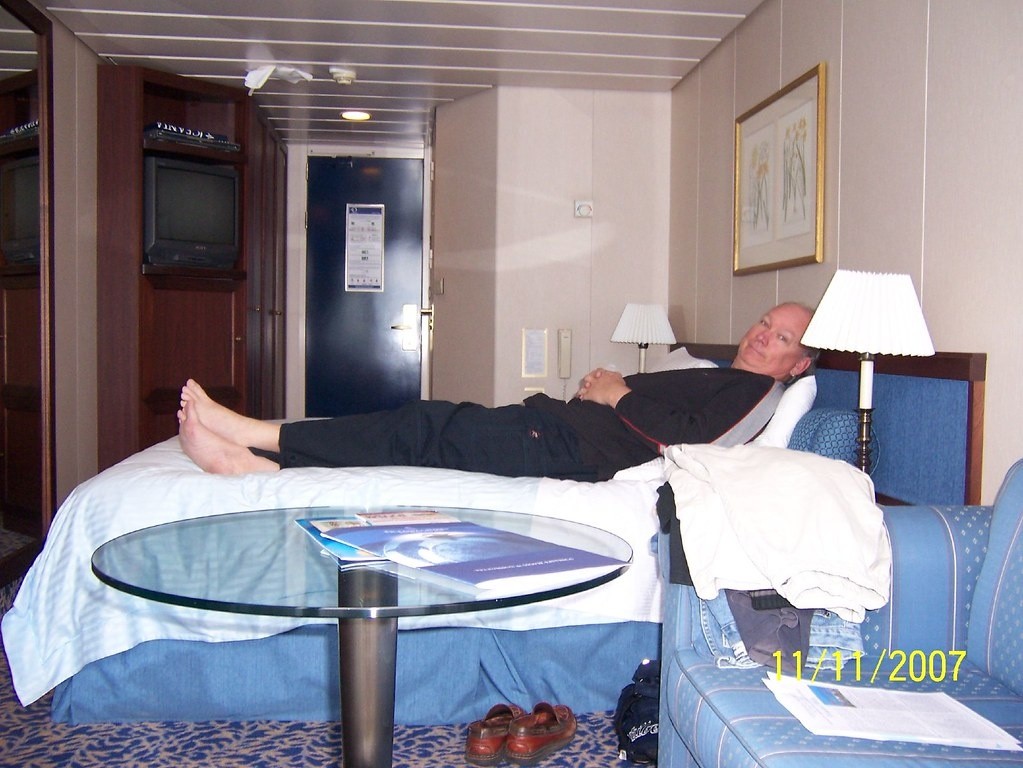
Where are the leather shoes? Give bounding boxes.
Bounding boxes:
[464,702,527,767]
[503,702,577,766]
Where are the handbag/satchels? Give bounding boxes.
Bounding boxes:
[614,685,660,764]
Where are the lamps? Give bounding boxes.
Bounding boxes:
[610,304,677,374]
[800,270,936,473]
[343,109,370,122]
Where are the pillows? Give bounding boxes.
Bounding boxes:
[647,346,818,447]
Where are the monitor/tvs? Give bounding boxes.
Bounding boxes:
[140,155,241,268]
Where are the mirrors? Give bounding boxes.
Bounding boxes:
[0,0,56,588]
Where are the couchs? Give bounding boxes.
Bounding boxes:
[657,459,1023,768]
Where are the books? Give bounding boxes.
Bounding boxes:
[294,510,634,598]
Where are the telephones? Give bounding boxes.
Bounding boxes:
[560,333,572,378]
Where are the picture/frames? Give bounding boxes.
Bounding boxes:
[733,63,826,277]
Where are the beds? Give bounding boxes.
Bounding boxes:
[3,343,986,725]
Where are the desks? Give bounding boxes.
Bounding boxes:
[91,506,634,768]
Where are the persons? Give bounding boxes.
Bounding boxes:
[177,301,819,482]
